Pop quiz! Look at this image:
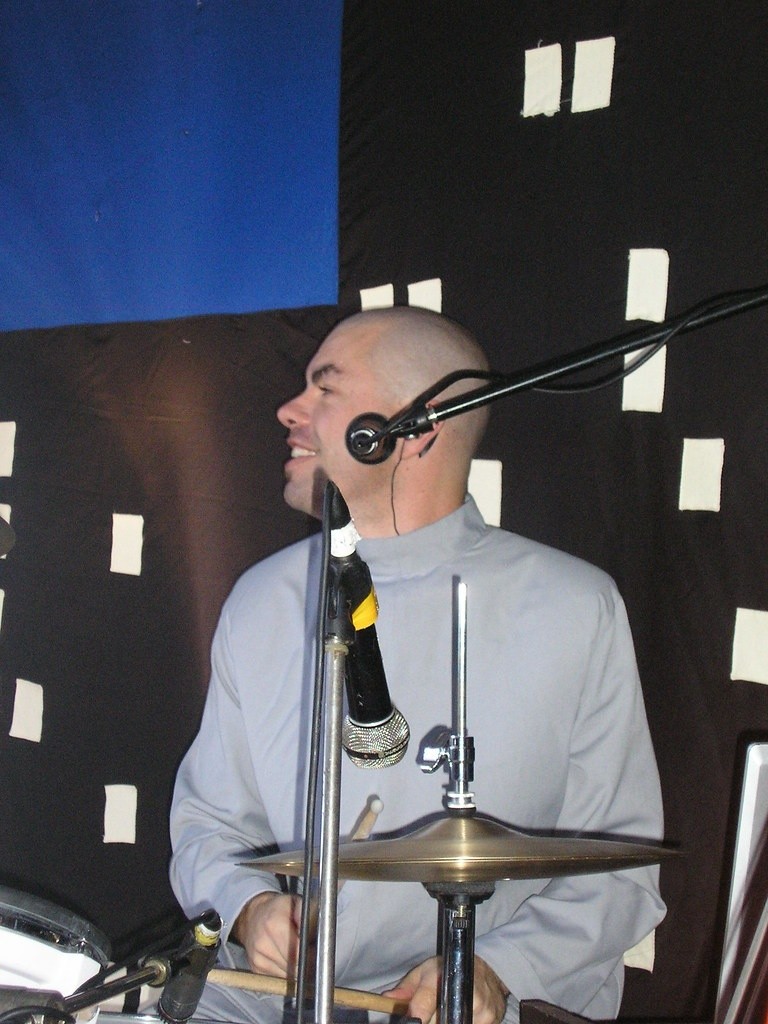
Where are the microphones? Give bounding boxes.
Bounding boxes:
[324,478,408,769]
[343,412,396,465]
[156,909,224,1024]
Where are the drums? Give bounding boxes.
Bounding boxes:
[0,879,118,1024]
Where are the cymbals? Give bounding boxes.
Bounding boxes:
[235,814,685,884]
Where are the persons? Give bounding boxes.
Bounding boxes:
[168,307,664,1024]
